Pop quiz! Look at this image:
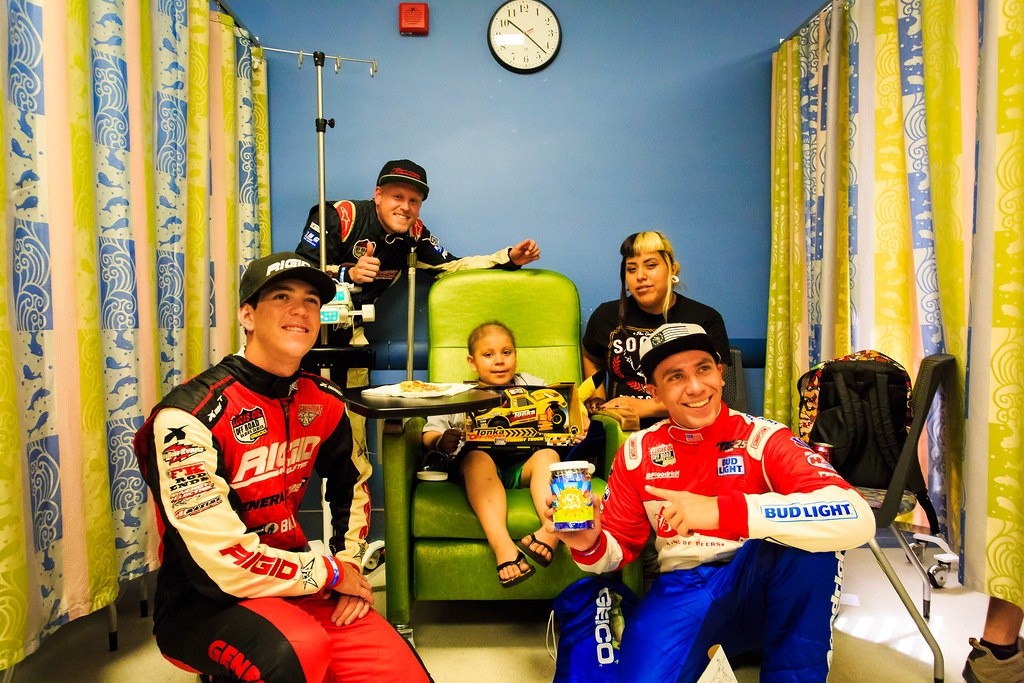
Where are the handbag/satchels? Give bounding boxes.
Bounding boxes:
[545,578,646,683]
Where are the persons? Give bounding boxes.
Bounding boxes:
[133,253,433,682]
[293,159,539,453]
[547,323,877,683]
[423,323,562,589]
[581,231,732,429]
[960,597,1024,683]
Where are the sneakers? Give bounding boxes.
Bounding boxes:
[960,637,1024,683]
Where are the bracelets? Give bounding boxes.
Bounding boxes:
[340,267,346,282]
[325,555,339,586]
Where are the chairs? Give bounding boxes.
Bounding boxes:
[855,351,955,683]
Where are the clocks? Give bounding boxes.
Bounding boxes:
[487,0,562,75]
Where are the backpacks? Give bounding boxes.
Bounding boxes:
[799,350,935,504]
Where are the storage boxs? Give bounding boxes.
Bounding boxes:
[462,380,584,447]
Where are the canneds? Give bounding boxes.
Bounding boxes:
[811,442,835,467]
[549,461,595,532]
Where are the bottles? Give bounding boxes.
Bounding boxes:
[550,460,596,532]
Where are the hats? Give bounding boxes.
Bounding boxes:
[240,251,337,308]
[636,325,717,382]
[377,160,430,202]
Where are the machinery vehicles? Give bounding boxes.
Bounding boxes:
[475,387,568,433]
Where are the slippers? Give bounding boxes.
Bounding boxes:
[517,536,552,569]
[497,553,536,587]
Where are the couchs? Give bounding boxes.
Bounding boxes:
[382,268,648,646]
